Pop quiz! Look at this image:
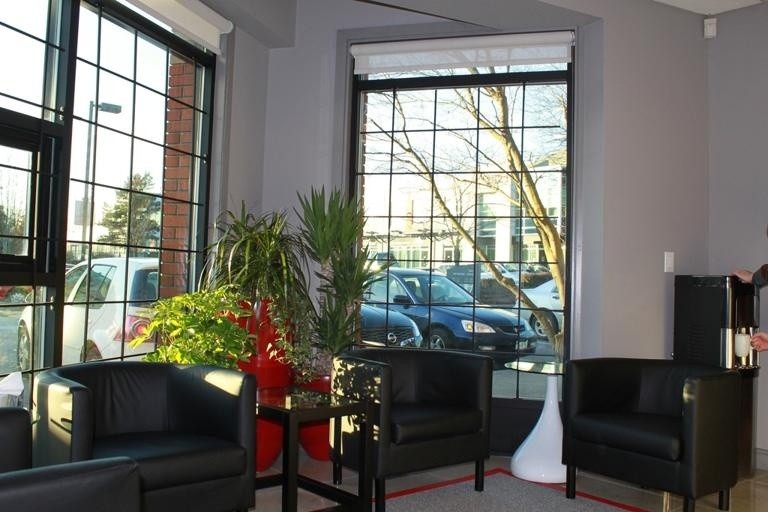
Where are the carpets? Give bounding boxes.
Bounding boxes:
[332,463,639,511]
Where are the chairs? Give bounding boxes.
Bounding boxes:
[331,343,495,512]
[560,345,746,512]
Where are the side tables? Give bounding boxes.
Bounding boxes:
[256,380,379,511]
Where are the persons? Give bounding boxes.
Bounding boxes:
[732,263,768,293]
[749,332,768,352]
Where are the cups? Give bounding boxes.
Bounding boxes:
[732,334,757,358]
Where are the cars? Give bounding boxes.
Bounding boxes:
[0,263,76,304]
[18,257,164,370]
[478,261,522,285]
[359,304,423,350]
[360,269,536,361]
[512,277,565,340]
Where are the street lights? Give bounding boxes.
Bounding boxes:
[79,100,121,260]
